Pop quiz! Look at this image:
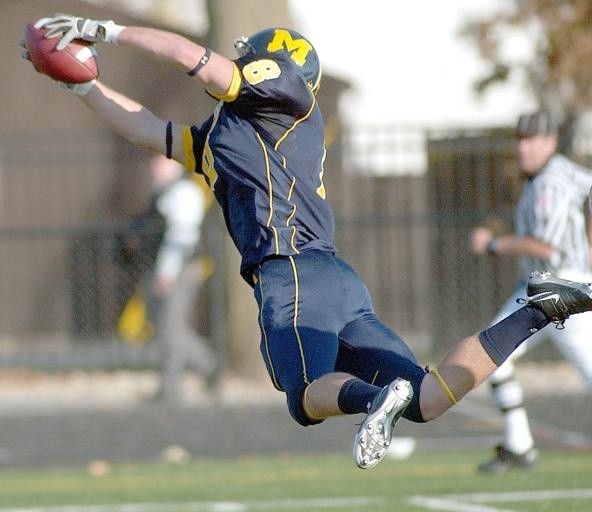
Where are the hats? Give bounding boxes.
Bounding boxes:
[511,114,556,136]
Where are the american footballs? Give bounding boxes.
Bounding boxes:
[24,17,99,83]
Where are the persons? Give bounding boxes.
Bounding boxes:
[59,204,162,346]
[469,109,590,475]
[141,153,223,406]
[17,12,589,470]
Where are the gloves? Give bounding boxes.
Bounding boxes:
[35,14,114,51]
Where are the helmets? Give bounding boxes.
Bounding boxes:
[234,29,322,98]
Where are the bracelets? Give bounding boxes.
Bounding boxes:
[486,234,499,258]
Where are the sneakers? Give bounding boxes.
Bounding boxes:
[352,376,414,470]
[479,444,540,473]
[517,270,592,329]
